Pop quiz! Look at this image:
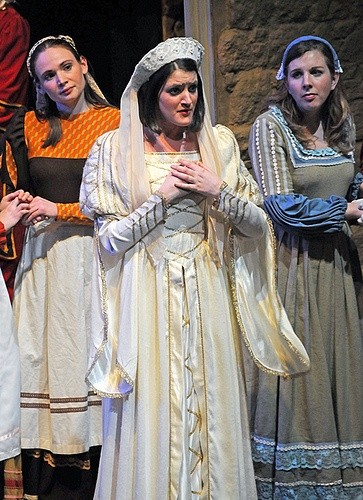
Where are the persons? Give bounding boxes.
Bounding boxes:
[79,37,313,500]
[246,35,363,500]
[0,0,120,500]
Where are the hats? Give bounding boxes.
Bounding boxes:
[130,37,205,93]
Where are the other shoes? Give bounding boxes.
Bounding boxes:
[58,466,82,490]
